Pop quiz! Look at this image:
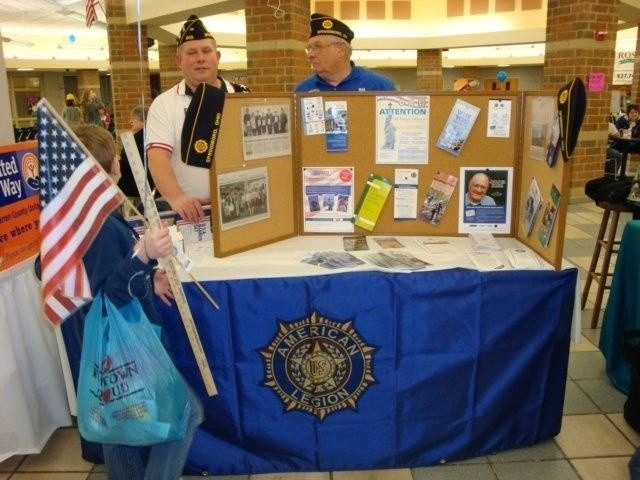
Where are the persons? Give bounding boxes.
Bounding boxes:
[221,182,270,221]
[55,86,148,204]
[326,108,336,132]
[294,13,397,95]
[142,14,253,225]
[28,125,203,480]
[465,172,496,208]
[607,104,640,176]
[380,105,396,150]
[242,109,288,137]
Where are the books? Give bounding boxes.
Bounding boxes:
[302,232,543,274]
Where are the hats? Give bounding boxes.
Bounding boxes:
[310,12,354,44]
[180,82,225,169]
[177,14,215,46]
[555,77,587,165]
[66,93,75,102]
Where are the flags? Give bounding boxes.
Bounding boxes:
[35,98,126,326]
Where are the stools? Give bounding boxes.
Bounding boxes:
[580,200,639,327]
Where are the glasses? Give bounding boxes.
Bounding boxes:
[305,45,322,56]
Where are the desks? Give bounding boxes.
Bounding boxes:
[60,222,579,474]
